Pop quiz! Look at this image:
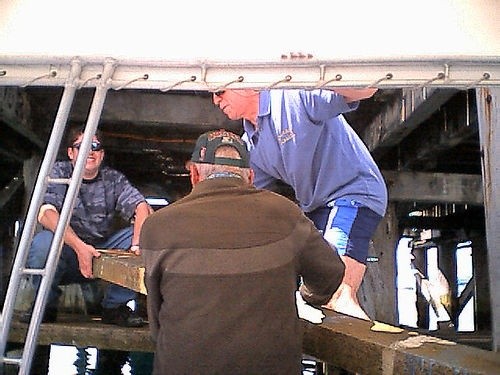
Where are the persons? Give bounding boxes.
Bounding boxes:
[24,125,154,328]
[140,130,347,375]
[213,52,387,320]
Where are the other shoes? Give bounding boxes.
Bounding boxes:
[20,307,58,323]
[102,308,143,327]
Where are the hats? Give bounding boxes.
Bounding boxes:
[185,128,250,170]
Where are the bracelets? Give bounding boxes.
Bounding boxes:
[131,245,139,251]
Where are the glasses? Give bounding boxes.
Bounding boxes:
[69,142,104,151]
[215,91,225,96]
